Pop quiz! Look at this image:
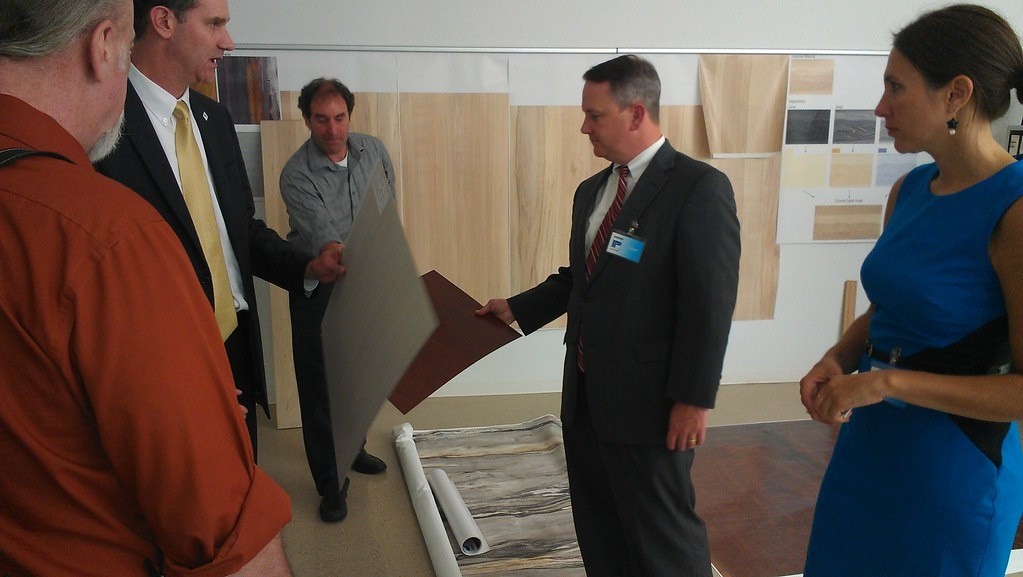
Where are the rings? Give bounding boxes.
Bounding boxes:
[817,393,823,402]
[687,439,697,442]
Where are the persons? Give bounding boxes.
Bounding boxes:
[0,0,292,577]
[798,3,1023,577]
[92,0,346,461]
[279,78,396,519]
[473,55,740,577]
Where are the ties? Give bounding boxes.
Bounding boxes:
[576,166,630,371]
[172,100,238,344]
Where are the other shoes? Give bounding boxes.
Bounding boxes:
[351,448,387,474]
[319,478,350,522]
